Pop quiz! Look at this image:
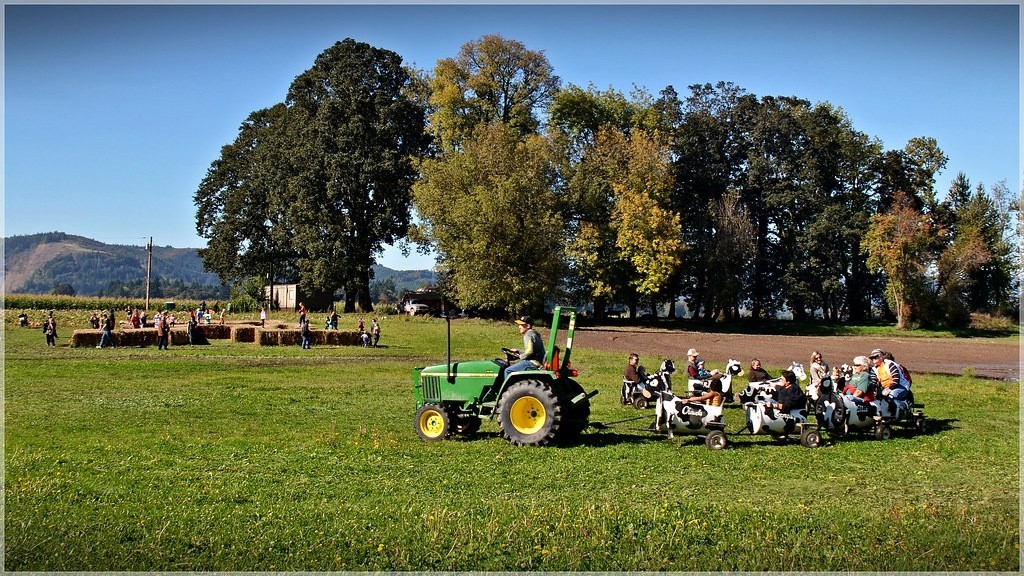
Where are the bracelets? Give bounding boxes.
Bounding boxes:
[777,404,779,408]
[688,399,690,402]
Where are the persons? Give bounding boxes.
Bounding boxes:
[683,346,721,381]
[260,308,265,328]
[361,331,369,347]
[218,309,225,324]
[504,316,545,381]
[325,312,342,330]
[746,357,776,382]
[19,311,28,327]
[41,311,59,347]
[89,308,118,350]
[226,302,235,314]
[832,349,912,417]
[370,318,381,348]
[681,379,724,407]
[154,307,176,351]
[196,301,221,321]
[124,305,148,329]
[358,317,365,331]
[810,351,830,384]
[625,353,652,390]
[298,302,307,327]
[186,308,198,345]
[300,320,314,348]
[763,368,808,415]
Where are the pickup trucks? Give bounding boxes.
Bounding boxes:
[396,299,429,316]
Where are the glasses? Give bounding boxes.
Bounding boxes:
[870,356,878,360]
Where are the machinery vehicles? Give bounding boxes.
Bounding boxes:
[414,305,598,448]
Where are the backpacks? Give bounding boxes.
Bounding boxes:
[110,320,114,330]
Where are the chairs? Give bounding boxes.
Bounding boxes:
[525,346,559,371]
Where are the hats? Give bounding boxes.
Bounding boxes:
[516,316,535,327]
[695,359,705,367]
[869,348,884,358]
[686,348,699,356]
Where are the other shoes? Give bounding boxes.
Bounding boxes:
[111,346,115,349]
[53,346,55,348]
[164,347,169,350]
[307,347,311,349]
[96,346,102,349]
[159,348,162,350]
[303,348,306,349]
